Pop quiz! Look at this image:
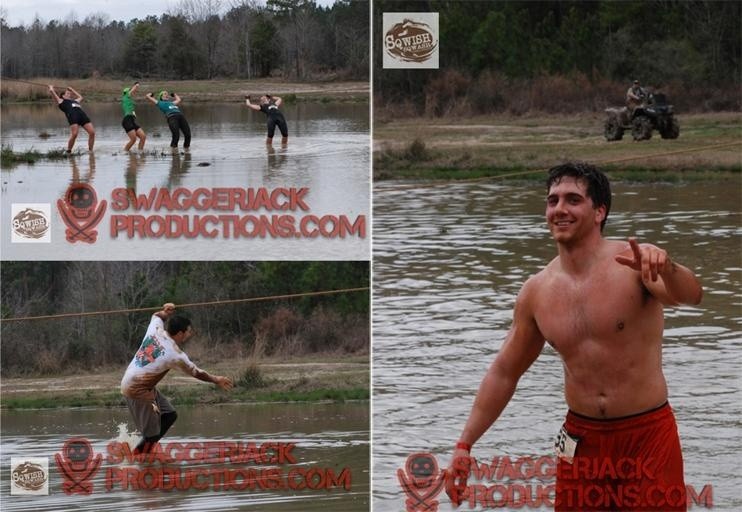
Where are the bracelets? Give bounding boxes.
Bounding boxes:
[456,442,472,453]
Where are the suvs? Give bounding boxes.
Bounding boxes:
[604,91,679,141]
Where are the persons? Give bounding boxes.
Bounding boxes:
[146,87,191,149]
[120,154,146,195]
[441,159,705,512]
[163,151,191,188]
[47,83,96,156]
[66,157,98,201]
[119,302,234,465]
[624,79,647,115]
[264,148,287,182]
[245,92,289,146]
[119,82,146,151]
